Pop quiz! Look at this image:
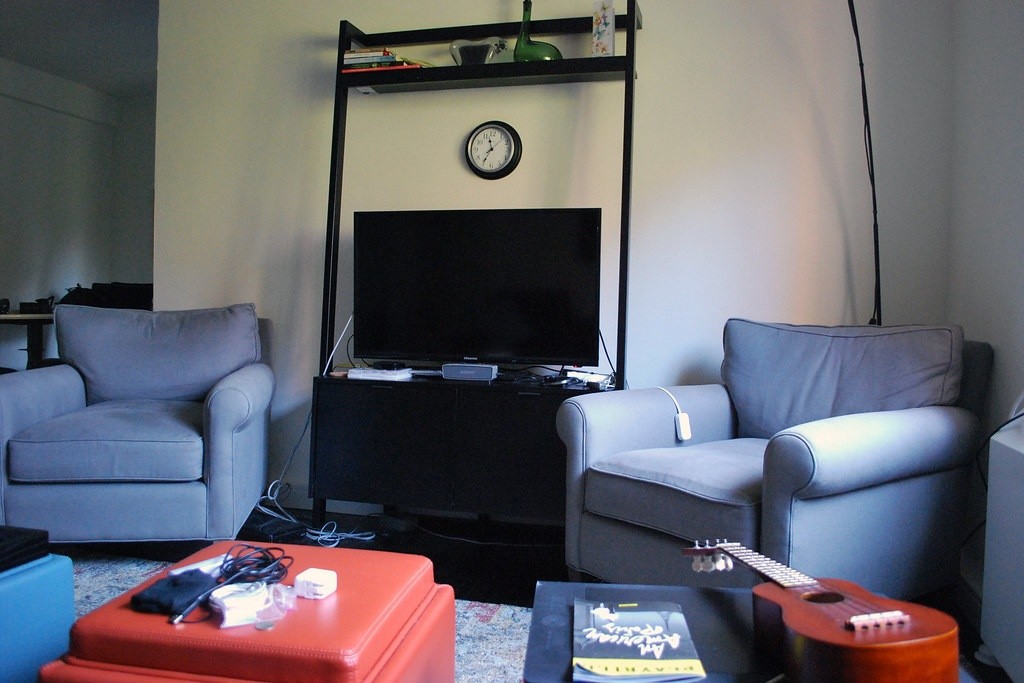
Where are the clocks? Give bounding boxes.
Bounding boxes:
[465,121,522,180]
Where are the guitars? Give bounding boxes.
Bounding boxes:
[681,539,959,683]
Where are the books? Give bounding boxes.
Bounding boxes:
[341,49,420,73]
[573,597,707,683]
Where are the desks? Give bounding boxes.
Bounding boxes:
[39,540,456,683]
[0,314,54,359]
[523,581,977,683]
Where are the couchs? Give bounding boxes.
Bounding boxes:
[0,302,274,543]
[555,317,994,604]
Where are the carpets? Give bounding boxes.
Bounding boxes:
[72,561,532,683]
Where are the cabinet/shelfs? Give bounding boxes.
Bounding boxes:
[307,0,643,528]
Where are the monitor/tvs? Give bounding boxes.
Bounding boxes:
[351,208,599,373]
